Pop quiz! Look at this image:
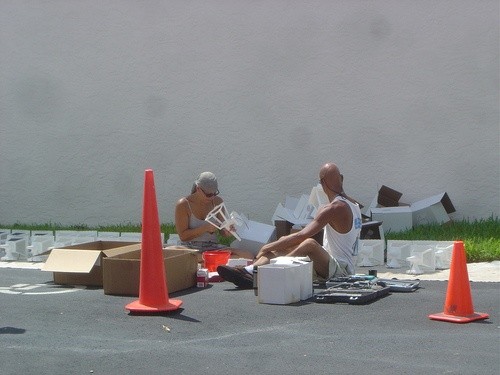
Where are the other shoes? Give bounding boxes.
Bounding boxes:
[216,263,254,289]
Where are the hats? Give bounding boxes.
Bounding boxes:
[196,172,219,193]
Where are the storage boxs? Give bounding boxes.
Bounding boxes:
[31,184,457,306]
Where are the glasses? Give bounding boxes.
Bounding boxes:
[199,187,219,197]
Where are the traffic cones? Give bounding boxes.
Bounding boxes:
[124,169,183,315]
[429,241,491,322]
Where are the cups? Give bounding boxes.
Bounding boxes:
[368,269,377,277]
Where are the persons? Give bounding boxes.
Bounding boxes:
[175,172,251,266]
[217,163,362,289]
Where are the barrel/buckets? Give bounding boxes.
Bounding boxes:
[202,250,232,271]
